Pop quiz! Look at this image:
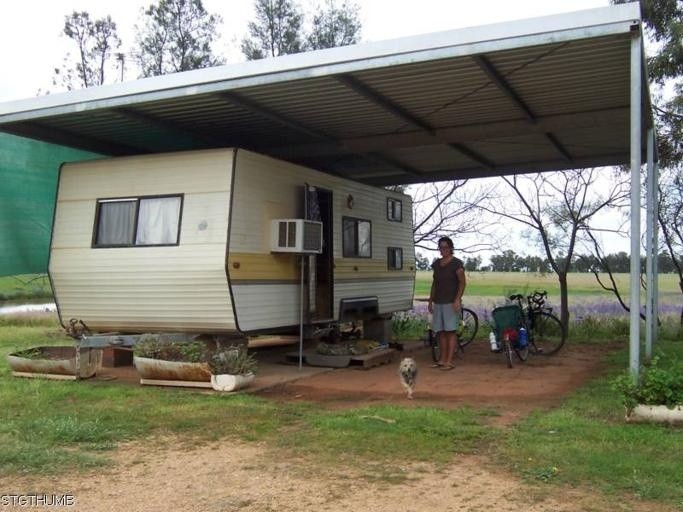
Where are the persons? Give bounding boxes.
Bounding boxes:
[427,237,466,372]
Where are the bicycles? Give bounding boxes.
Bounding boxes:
[428,305,479,363]
[489,290,566,368]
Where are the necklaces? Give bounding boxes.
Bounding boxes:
[441,257,450,265]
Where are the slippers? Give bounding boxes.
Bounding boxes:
[429,363,456,370]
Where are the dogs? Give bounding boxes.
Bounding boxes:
[399,356,418,400]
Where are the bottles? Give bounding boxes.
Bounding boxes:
[489,332,497,351]
[519,327,527,345]
